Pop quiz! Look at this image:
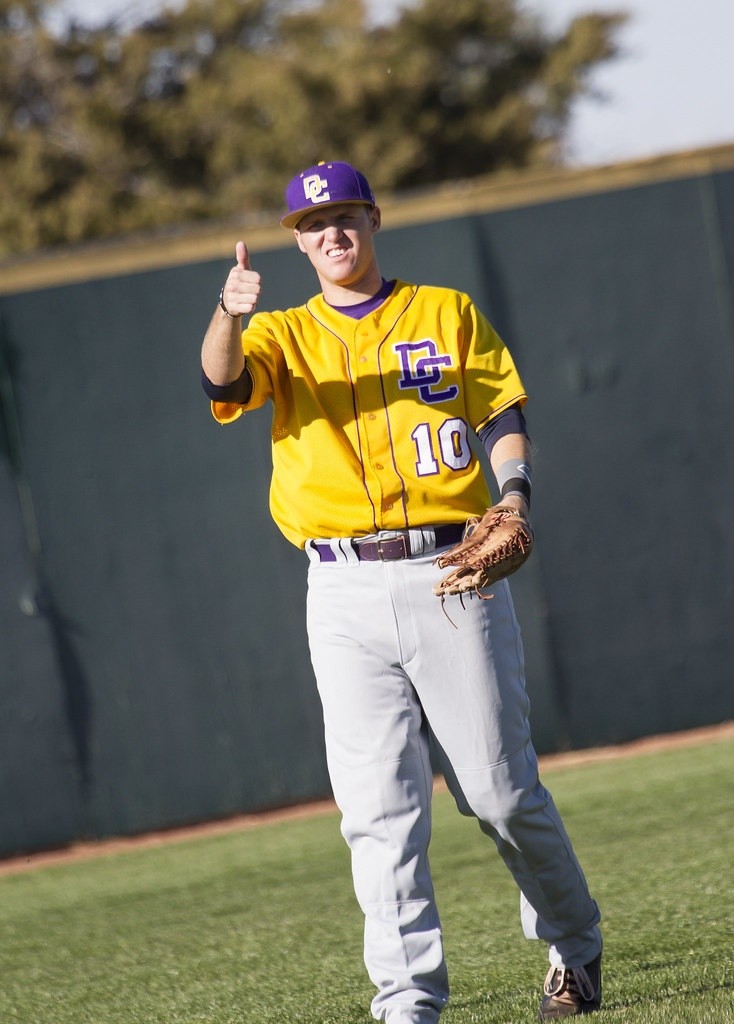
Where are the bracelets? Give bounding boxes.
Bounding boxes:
[218,287,245,320]
[496,459,532,513]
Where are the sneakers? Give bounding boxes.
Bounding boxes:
[539,952,601,1017]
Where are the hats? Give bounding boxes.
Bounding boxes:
[281,160,375,230]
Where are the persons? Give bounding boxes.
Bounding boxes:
[199,164,607,1023]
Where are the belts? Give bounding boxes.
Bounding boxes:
[310,523,464,562]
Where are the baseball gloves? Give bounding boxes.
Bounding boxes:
[432,506,534,596]
